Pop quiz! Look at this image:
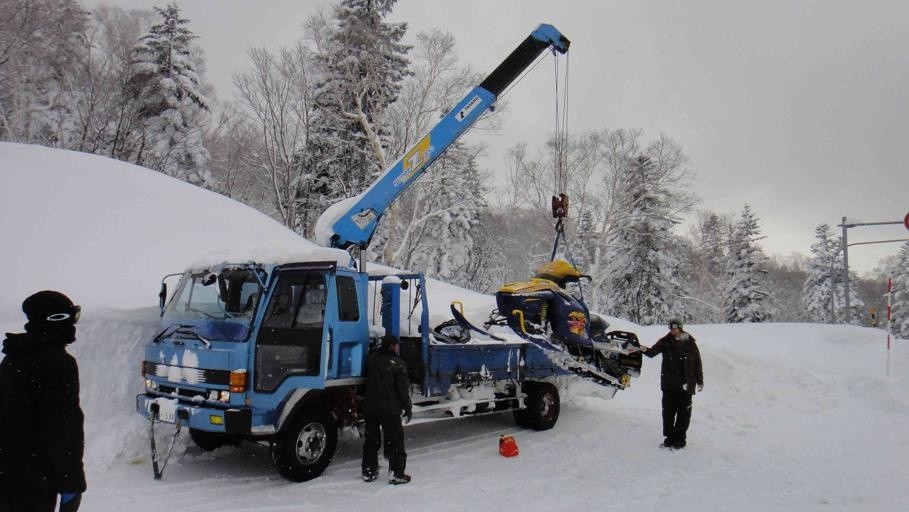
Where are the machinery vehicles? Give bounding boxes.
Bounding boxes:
[132,18,577,484]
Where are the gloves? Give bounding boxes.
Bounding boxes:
[403,410,412,424]
[59,492,82,511]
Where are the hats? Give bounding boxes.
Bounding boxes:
[381,335,402,345]
[668,319,684,329]
[22,290,74,324]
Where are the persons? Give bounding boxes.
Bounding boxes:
[360,336,415,485]
[1,290,88,512]
[640,318,705,450]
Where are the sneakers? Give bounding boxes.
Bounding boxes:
[659,443,685,451]
[362,473,377,481]
[389,475,411,484]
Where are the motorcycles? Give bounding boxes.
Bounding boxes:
[450,254,643,390]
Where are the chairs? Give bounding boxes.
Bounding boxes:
[242,277,325,328]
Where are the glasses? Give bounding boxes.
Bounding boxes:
[43,305,81,325]
